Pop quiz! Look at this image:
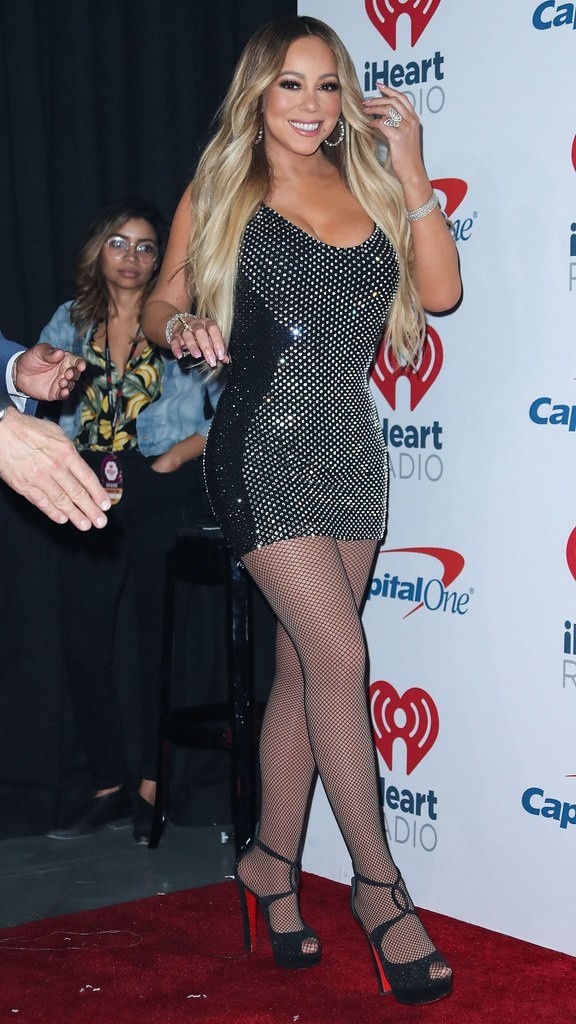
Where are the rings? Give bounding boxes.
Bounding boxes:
[382,107,403,129]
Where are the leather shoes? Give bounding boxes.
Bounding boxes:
[131,791,167,846]
[47,784,131,839]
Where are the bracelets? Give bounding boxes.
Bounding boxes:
[165,313,201,358]
[404,189,440,222]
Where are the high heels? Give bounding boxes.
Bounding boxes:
[234,822,321,970]
[348,860,456,1004]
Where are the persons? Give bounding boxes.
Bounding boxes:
[0,15,454,1006]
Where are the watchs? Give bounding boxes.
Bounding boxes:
[0,408,6,421]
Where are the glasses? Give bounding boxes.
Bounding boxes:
[105,236,158,263]
